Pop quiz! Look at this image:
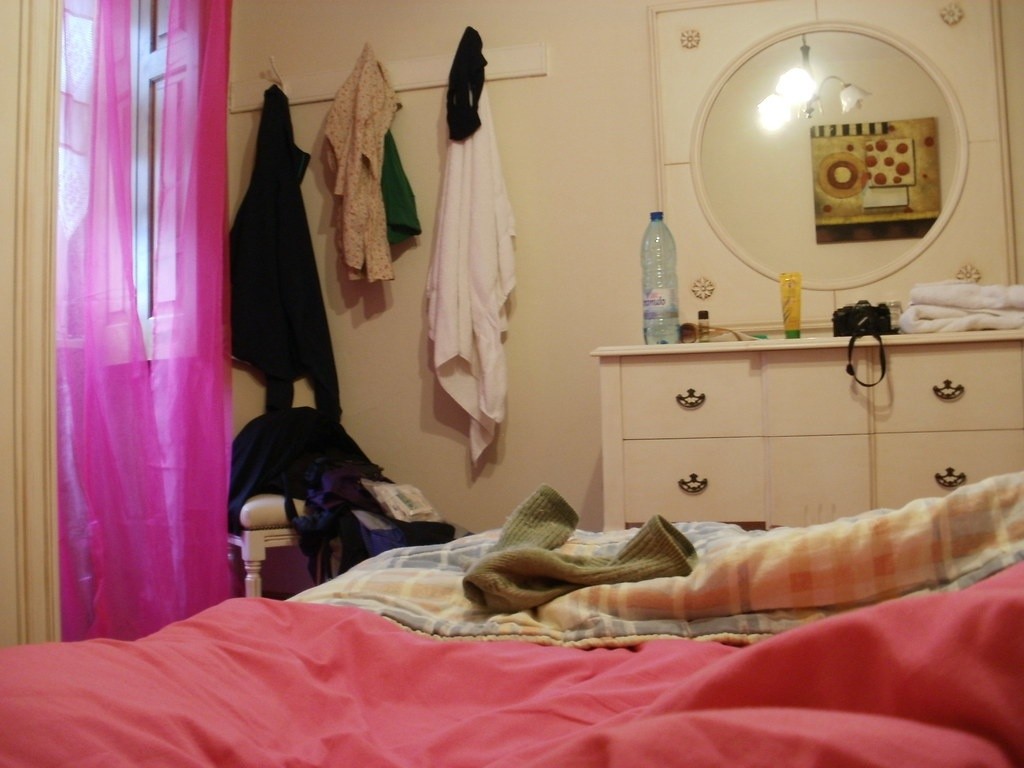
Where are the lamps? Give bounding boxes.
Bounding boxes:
[758,35,866,130]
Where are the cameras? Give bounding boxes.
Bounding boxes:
[833,299,891,338]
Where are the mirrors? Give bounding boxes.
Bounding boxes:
[648,0,1014,332]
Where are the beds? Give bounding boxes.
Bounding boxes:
[0,471,1024,767]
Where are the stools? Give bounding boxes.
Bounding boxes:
[229,492,304,598]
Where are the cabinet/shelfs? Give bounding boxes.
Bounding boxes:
[590,328,1024,533]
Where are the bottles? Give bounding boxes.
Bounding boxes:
[641,211,680,344]
[698,310,708,342]
